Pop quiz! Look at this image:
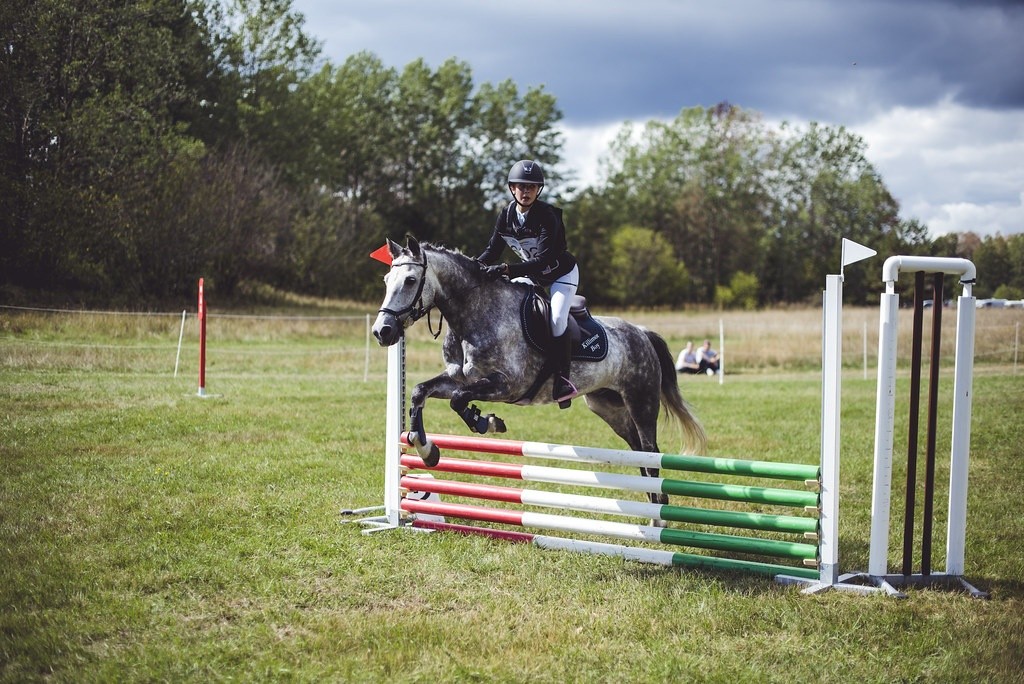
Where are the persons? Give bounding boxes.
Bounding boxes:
[477,160,579,400]
[675,341,699,372]
[696,341,720,376]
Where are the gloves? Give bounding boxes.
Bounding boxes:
[480,265,506,282]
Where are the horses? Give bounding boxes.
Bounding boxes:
[370,233,707,528]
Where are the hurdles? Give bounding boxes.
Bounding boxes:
[345,235,889,602]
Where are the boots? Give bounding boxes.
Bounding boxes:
[550,317,574,410]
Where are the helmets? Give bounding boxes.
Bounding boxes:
[508,160,544,186]
